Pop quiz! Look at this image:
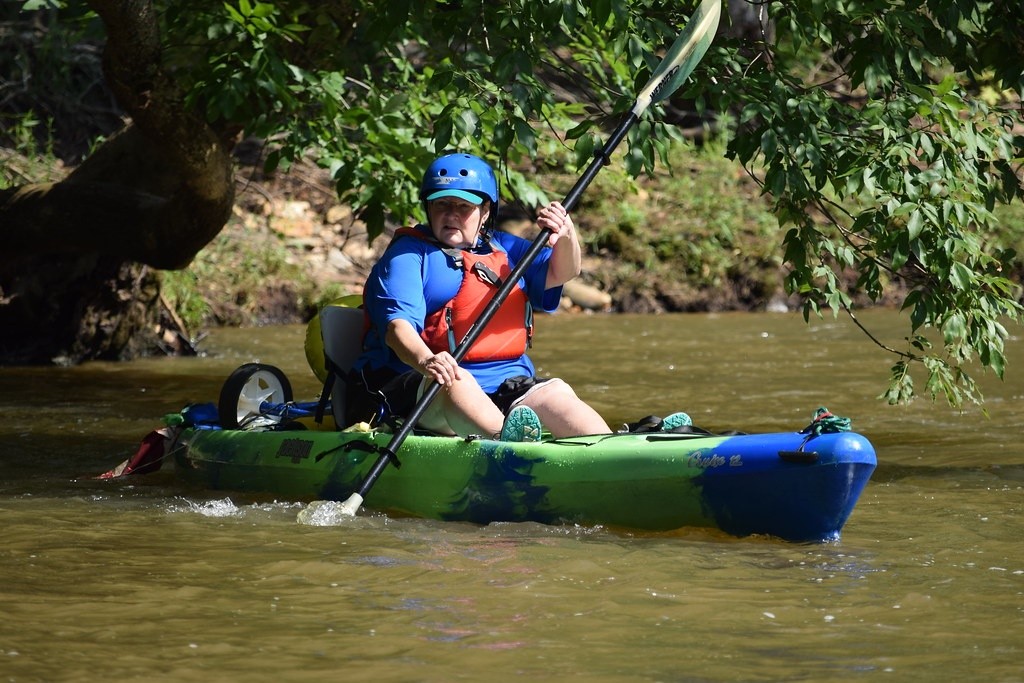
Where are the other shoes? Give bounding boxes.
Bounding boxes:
[654,411,692,431]
[492,405,542,443]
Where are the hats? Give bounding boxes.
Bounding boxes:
[423,188,484,205]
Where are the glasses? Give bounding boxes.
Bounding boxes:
[426,199,485,212]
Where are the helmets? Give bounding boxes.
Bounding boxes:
[420,152,499,216]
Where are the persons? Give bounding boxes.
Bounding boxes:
[350,153,692,443]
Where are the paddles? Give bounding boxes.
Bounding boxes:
[341,0,722,517]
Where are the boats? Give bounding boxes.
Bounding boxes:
[102,406,876,544]
